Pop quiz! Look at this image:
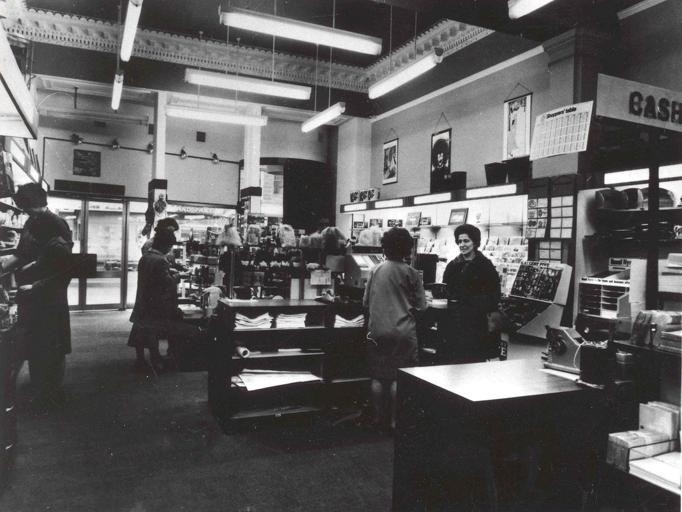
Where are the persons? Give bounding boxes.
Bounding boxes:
[15,210,75,412]
[362,224,434,438]
[140,216,192,366]
[431,138,449,191]
[13,182,75,389]
[436,223,502,364]
[126,227,189,374]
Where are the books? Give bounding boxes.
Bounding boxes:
[414,234,528,298]
[657,328,681,355]
[607,401,681,497]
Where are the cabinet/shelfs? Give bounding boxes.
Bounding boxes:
[208,299,440,434]
[0,199,29,379]
[394,359,637,512]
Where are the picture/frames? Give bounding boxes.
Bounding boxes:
[448,208,468,225]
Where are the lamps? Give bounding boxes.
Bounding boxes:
[164,104,267,127]
[218,4,383,58]
[507,0,556,20]
[368,45,443,101]
[112,69,125,112]
[119,0,144,62]
[300,101,347,133]
[184,67,312,101]
